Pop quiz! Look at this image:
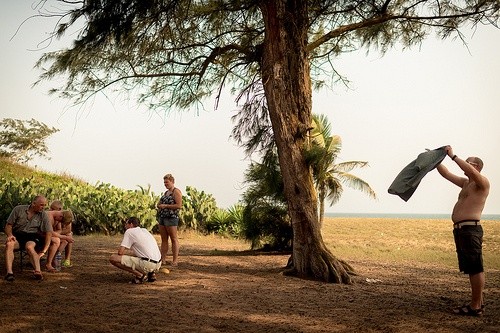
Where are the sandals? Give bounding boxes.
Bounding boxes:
[449,304,482,316]
[129,274,148,284]
[148,271,156,281]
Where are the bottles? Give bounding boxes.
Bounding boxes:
[55,251,62,271]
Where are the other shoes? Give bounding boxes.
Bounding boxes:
[6,273,15,280]
[62,259,71,266]
[33,271,42,279]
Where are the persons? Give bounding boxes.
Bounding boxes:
[46,201,74,272]
[157,174,182,267]
[425,145,491,316]
[110,217,162,284]
[5,196,53,281]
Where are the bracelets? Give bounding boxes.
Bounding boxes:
[40,251,47,254]
[452,155,457,160]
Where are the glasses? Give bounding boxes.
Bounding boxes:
[467,161,479,166]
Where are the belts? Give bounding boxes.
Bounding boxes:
[453,222,480,228]
[141,257,162,264]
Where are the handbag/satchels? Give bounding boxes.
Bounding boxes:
[44,265,59,273]
